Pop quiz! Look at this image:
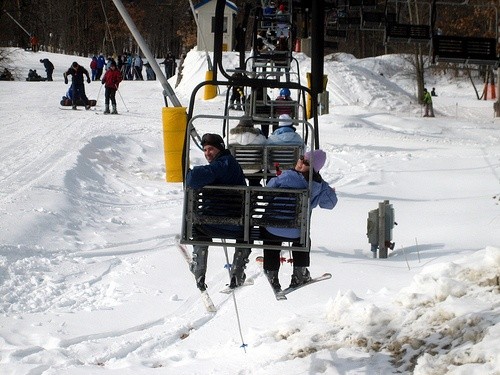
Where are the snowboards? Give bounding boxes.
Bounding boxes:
[60,99,97,106]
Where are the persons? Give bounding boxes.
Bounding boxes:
[31,35,38,52]
[4,68,11,80]
[431,88,437,96]
[160,52,186,87]
[90,55,116,81]
[117,53,156,80]
[223,115,266,187]
[40,58,54,81]
[187,133,257,290]
[102,61,122,114]
[231,68,243,110]
[260,149,338,288]
[63,62,91,110]
[272,89,296,131]
[422,88,434,117]
[28,69,42,80]
[247,87,271,138]
[265,114,305,184]
[253,0,293,81]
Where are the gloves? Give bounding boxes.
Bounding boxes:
[87,78,90,83]
[65,79,68,84]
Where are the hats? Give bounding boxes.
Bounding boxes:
[239,115,254,126]
[201,133,225,151]
[279,114,293,126]
[304,149,326,172]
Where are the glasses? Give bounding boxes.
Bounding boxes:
[299,155,310,167]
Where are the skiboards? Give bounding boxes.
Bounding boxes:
[255,255,333,302]
[172,233,255,313]
[89,109,125,115]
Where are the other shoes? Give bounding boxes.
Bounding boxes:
[111,110,117,114]
[229,272,246,288]
[290,268,313,287]
[197,283,209,291]
[72,106,77,110]
[104,108,110,114]
[85,105,90,110]
[267,278,283,292]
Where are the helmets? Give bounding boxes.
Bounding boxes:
[280,88,290,96]
